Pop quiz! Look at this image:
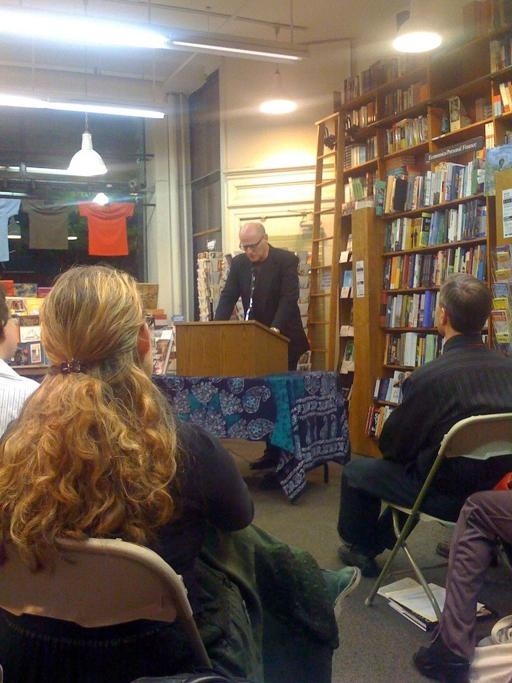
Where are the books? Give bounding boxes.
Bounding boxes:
[448,95,468,131]
[386,115,428,156]
[364,406,397,439]
[493,268,512,286]
[372,370,413,403]
[383,244,486,291]
[441,104,450,133]
[340,270,354,298]
[474,97,494,122]
[341,301,353,336]
[343,135,379,167]
[490,35,512,71]
[344,52,408,99]
[345,99,378,131]
[387,583,499,632]
[492,296,510,311]
[494,251,511,266]
[379,291,440,328]
[383,331,445,367]
[374,162,484,216]
[385,200,487,252]
[495,244,512,257]
[465,1,512,38]
[384,81,430,115]
[337,275,512,576]
[343,172,376,206]
[492,81,512,115]
[341,339,354,372]
[491,281,511,297]
[492,309,511,345]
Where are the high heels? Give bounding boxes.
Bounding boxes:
[412,633,471,683]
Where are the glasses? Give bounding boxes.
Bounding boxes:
[239,236,264,250]
[145,319,156,329]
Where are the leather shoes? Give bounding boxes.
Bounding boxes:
[434,539,450,557]
[319,565,362,622]
[337,543,381,578]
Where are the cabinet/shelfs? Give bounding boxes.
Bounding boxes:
[332,21,511,460]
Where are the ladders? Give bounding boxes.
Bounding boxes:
[307,110,346,371]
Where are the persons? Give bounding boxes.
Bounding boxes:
[0,289,41,445]
[411,490,512,683]
[215,224,312,372]
[0,265,361,683]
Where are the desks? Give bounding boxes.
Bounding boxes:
[150,319,338,485]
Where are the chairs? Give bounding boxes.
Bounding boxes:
[356,410,512,631]
[0,535,228,683]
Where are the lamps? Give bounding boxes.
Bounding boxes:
[253,28,301,118]
[387,0,445,55]
[0,91,168,120]
[7,223,22,240]
[171,2,310,63]
[63,0,108,176]
[92,189,110,206]
